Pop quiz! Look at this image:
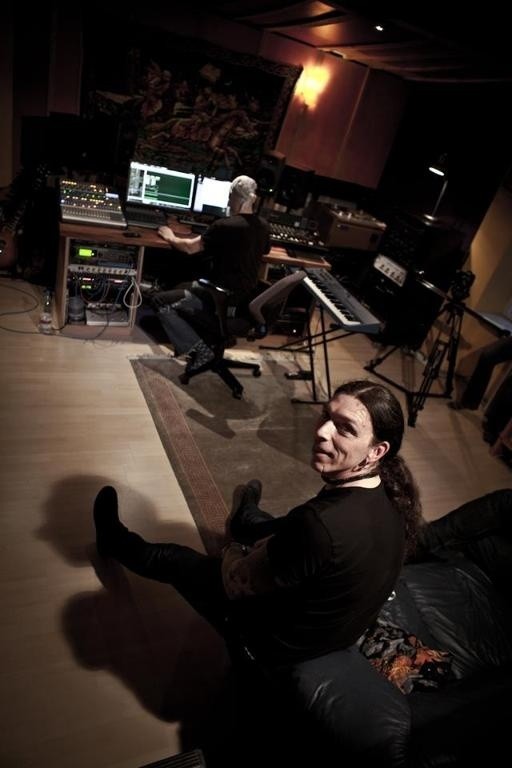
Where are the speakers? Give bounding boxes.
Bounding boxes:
[273,156,314,210]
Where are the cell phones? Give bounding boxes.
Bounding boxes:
[123,232,141,238]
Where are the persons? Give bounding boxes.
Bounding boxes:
[93,380,422,668]
[150,173,270,371]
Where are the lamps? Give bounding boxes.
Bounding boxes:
[424,148,453,222]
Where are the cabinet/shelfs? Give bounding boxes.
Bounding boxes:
[314,201,387,290]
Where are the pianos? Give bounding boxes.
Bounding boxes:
[295,267,380,334]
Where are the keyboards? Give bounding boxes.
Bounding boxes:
[124,206,167,230]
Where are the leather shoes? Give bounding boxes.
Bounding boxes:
[231,479,262,538]
[92,486,128,559]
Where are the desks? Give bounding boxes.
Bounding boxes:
[51,218,331,346]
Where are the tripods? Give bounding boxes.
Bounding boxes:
[365,299,466,431]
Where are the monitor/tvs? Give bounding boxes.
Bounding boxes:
[193,172,234,218]
[125,158,198,215]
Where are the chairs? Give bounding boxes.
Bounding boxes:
[178,267,305,403]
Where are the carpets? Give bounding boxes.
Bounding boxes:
[123,354,332,567]
